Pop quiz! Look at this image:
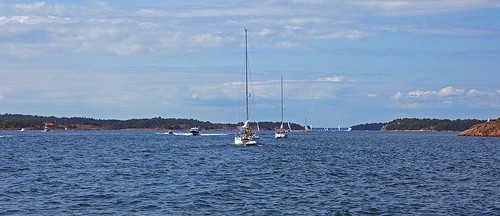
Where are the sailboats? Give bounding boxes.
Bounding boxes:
[304,117,313,132]
[274,77,289,139]
[233,27,259,147]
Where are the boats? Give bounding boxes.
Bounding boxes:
[43,121,54,131]
[189,127,200,136]
